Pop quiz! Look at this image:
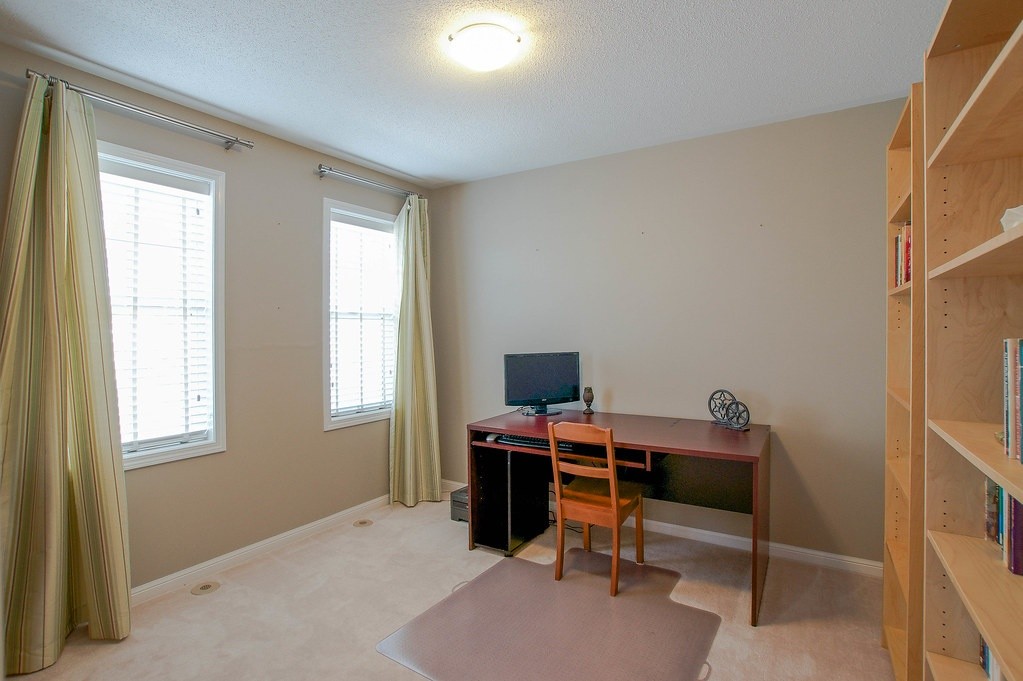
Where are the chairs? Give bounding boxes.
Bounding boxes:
[546,420,644,596]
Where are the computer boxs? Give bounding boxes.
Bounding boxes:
[472,445,550,552]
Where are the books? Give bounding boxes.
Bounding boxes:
[979,634,1006,681]
[984,476,1023,577]
[1002,339,1023,465]
[894,221,911,288]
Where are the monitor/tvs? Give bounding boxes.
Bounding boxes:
[504,351,580,416]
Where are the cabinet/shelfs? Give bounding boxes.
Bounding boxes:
[884,0,1023,681]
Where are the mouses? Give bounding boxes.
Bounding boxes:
[486,434,502,441]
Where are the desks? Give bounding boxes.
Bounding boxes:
[464,407,770,630]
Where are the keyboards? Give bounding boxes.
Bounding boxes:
[498,434,575,451]
